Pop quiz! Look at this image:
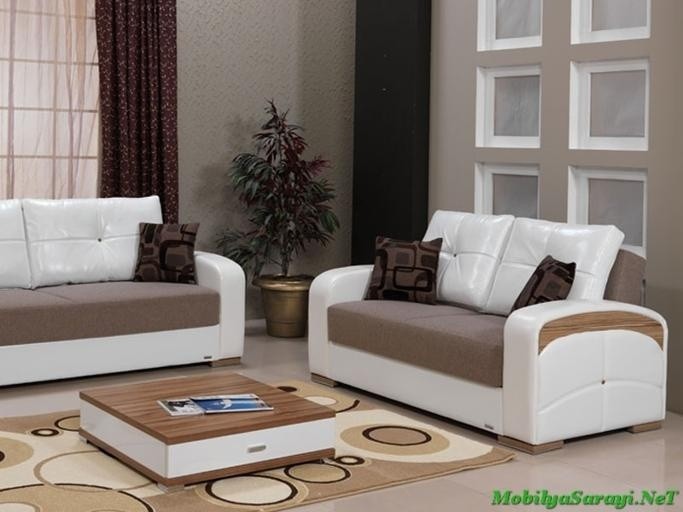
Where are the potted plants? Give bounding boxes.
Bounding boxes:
[214,96,341,338]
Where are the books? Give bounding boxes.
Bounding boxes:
[187,393,275,414]
[156,397,205,417]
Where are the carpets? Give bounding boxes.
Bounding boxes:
[0,379,515,512]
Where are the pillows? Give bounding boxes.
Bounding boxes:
[363,236,443,304]
[133,223,200,284]
[507,255,576,317]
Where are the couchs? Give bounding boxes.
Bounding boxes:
[308,209,668,455]
[0,195,246,387]
